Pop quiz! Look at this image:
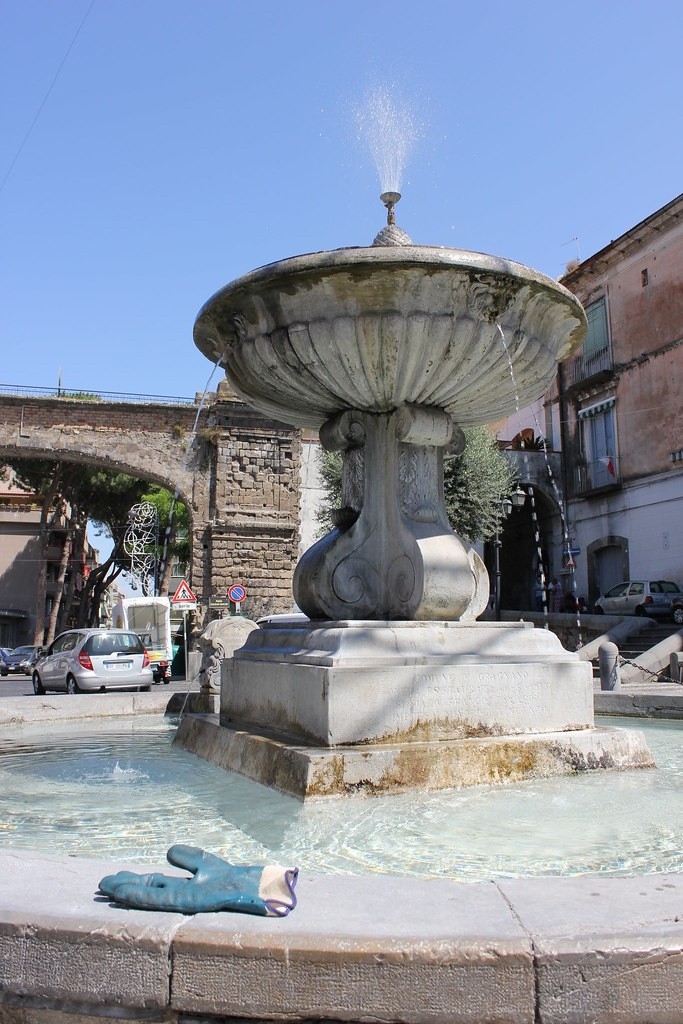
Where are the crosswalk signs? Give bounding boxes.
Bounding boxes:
[171,580,197,602]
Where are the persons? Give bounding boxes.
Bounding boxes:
[531,578,547,612]
[548,577,562,614]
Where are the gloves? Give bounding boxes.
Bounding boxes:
[99,845,299,917]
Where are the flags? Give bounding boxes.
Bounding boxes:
[598,458,615,478]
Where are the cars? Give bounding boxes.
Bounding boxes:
[32,628,153,696]
[594,579,682,617]
[1,646,46,676]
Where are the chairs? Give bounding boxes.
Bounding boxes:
[650,588,656,593]
[630,590,637,595]
[100,637,113,649]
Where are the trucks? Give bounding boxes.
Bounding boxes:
[112,597,173,684]
